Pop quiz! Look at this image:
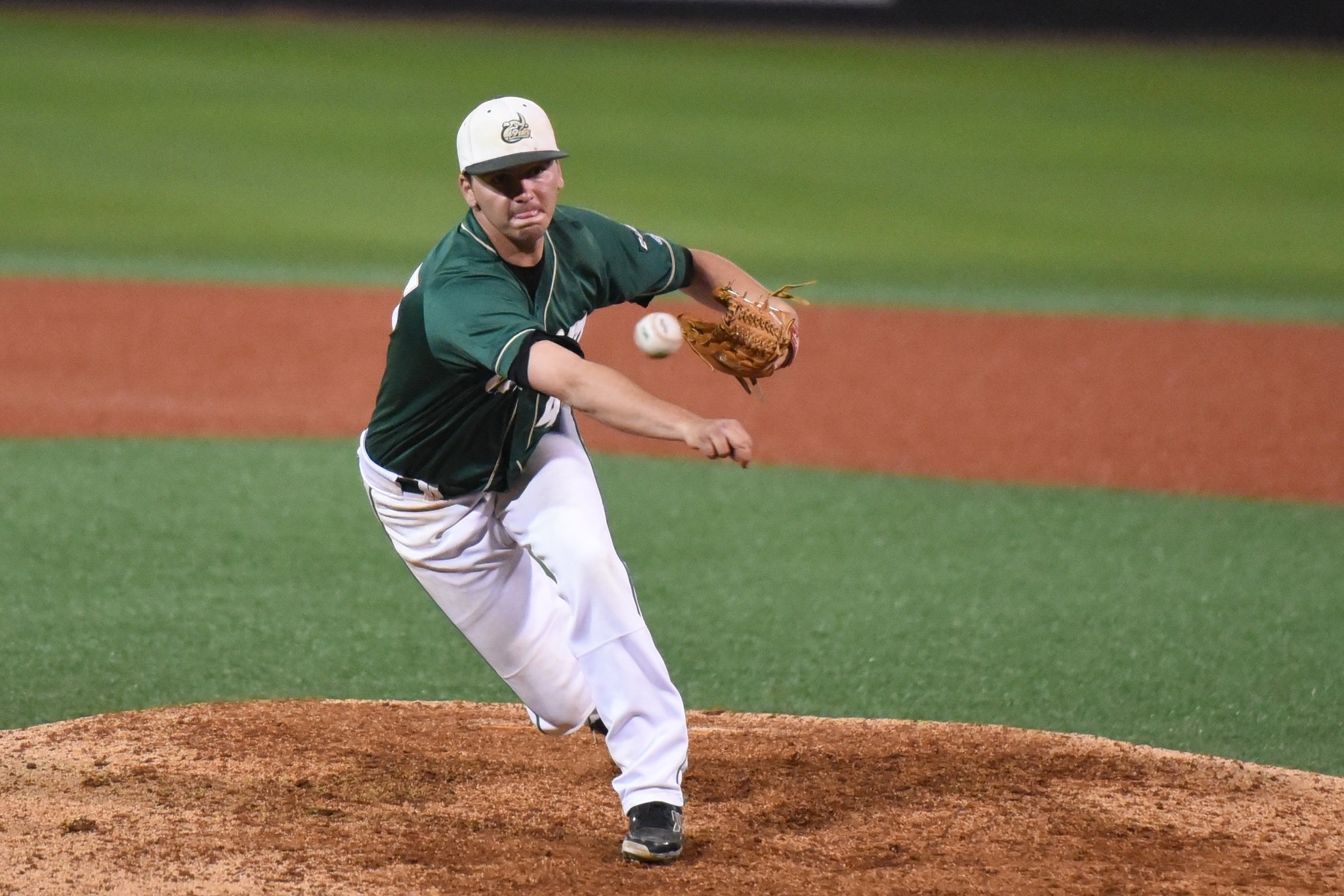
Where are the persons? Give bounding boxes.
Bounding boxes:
[357,96,800,862]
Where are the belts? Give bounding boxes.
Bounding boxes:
[397,476,465,498]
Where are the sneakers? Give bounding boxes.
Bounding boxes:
[588,716,607,743]
[620,803,685,861]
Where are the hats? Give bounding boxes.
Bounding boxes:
[455,97,569,175]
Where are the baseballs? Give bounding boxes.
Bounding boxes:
[635,312,685,360]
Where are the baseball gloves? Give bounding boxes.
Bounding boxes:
[676,278,821,396]
